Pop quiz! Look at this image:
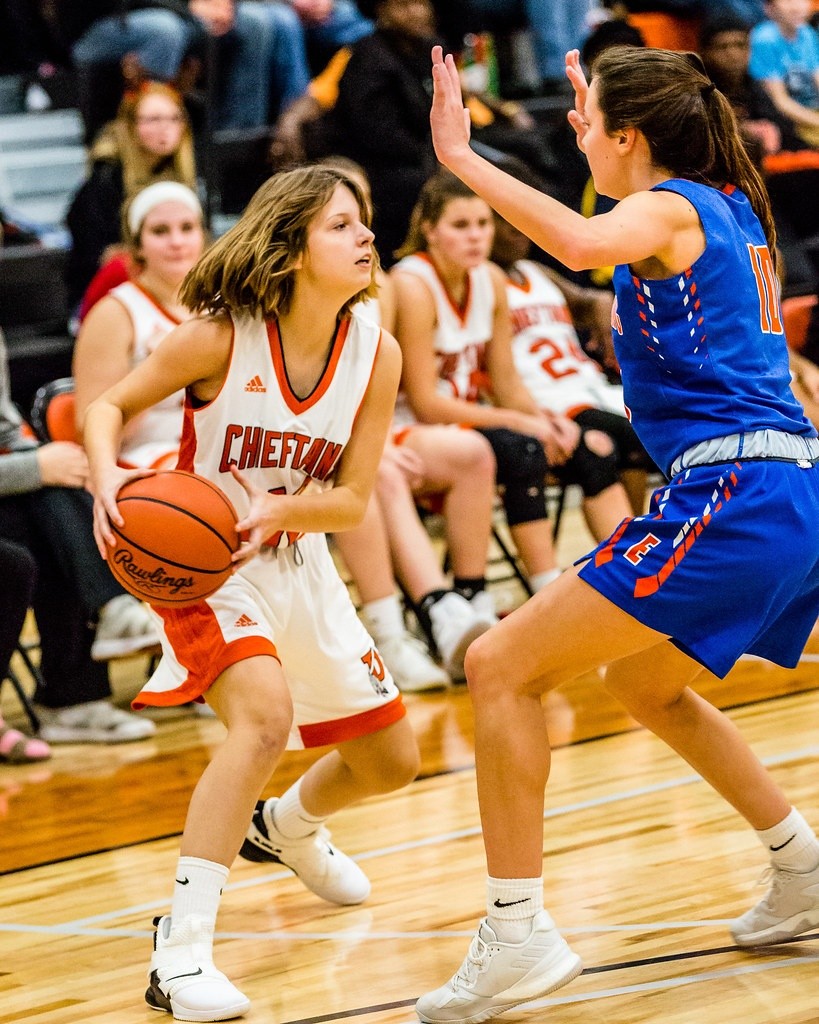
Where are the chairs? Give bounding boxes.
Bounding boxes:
[211,96,574,212]
[777,295,819,353]
[0,376,662,736]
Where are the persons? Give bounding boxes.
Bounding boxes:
[82,163,422,1023]
[415,45,819,1024]
[0,0,819,764]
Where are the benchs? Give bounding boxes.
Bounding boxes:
[0,216,257,358]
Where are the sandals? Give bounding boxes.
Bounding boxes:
[0,725,51,764]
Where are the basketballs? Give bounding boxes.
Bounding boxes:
[103,468,241,610]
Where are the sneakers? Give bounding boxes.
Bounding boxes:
[428,591,502,684]
[145,914,250,1022]
[377,631,449,692]
[730,859,819,947]
[415,910,583,1024]
[92,597,162,661]
[237,797,371,906]
[40,701,158,744]
